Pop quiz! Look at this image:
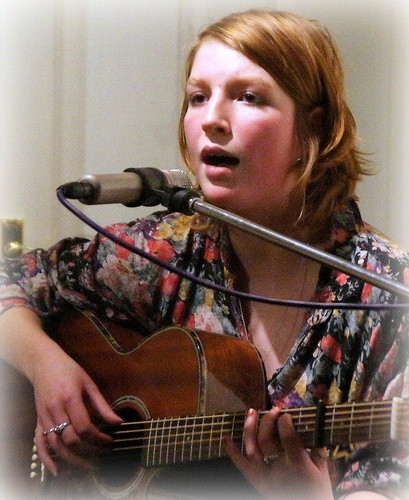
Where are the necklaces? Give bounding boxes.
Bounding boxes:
[242,245,303,362]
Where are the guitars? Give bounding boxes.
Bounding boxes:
[0,302,409,499]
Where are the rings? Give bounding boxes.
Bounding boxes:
[54,421,72,434]
[43,426,56,435]
[262,453,279,467]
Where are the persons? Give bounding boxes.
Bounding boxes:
[0,8,409,500]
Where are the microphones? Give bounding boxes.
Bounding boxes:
[57,169,193,208]
